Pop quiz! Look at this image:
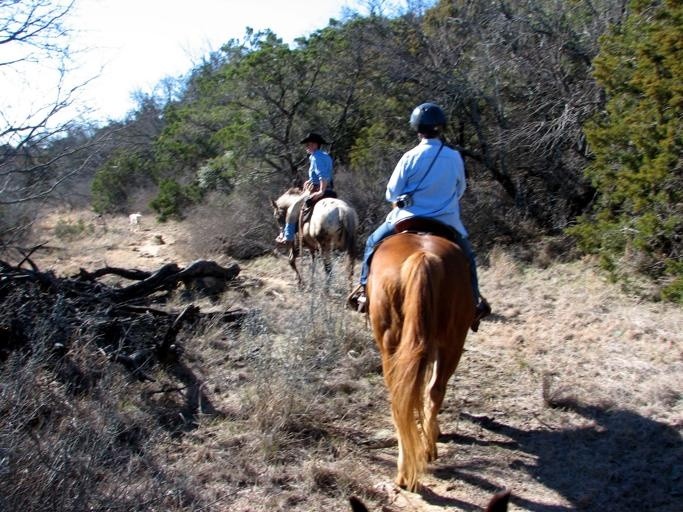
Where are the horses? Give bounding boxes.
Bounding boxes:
[270,187,360,293]
[365,203,474,493]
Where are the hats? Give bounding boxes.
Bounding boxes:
[300,132,330,145]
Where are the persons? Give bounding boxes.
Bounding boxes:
[349,102,484,333]
[281,132,333,244]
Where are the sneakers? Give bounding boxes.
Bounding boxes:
[471,301,492,332]
[346,291,369,314]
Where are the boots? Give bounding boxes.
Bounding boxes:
[276,223,297,243]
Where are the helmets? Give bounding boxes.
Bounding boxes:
[409,103,446,132]
